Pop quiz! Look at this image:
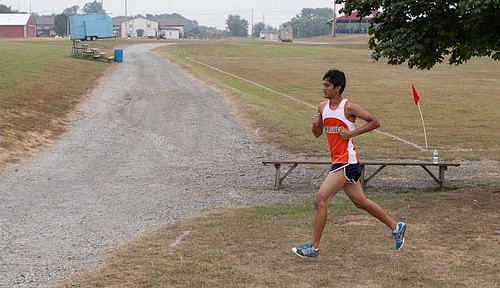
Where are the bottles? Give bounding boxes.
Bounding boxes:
[432,147,438,164]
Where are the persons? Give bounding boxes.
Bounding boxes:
[292,70,406,258]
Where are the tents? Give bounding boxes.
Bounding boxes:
[325,9,383,34]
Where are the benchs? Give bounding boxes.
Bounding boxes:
[262,159,460,191]
[72,39,114,62]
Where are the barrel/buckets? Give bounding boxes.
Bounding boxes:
[114,50,123,62]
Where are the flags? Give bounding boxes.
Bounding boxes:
[412,83,421,107]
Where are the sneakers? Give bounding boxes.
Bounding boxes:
[391,222,407,251]
[292,242,319,259]
